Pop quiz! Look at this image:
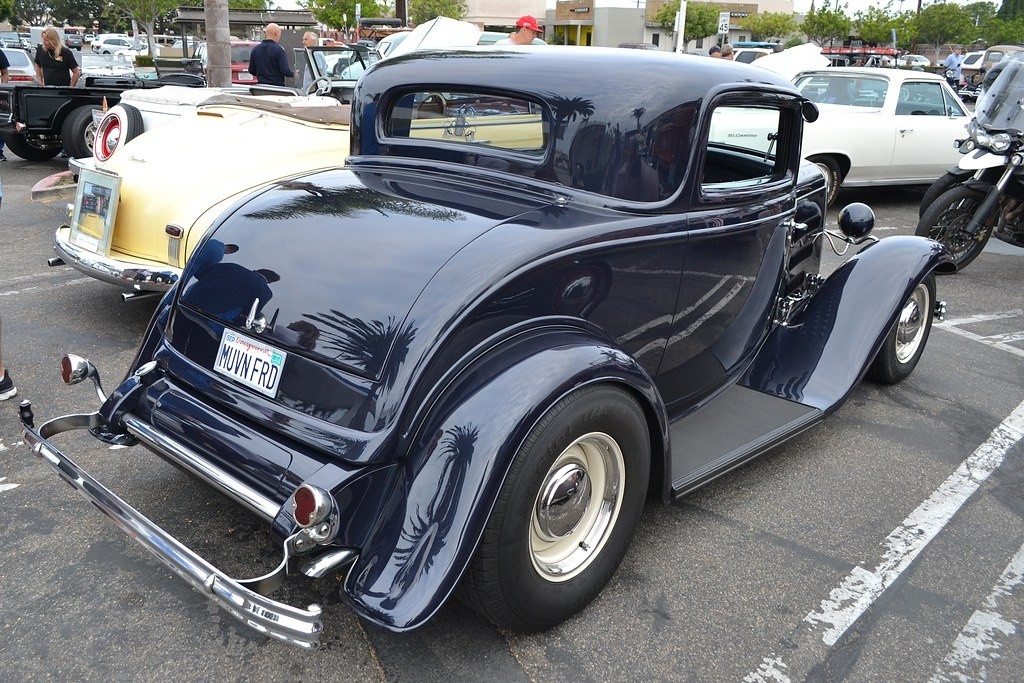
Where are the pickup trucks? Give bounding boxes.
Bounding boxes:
[0,57,208,160]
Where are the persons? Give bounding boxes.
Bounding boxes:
[721,44,734,62]
[181,261,282,331]
[248,23,300,87]
[493,15,545,46]
[182,233,241,269]
[773,45,784,53]
[286,319,321,353]
[709,45,721,58]
[943,45,963,90]
[300,31,328,93]
[0,49,11,162]
[34,28,79,86]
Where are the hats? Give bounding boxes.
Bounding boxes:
[709,46,721,55]
[516,15,544,33]
[722,44,733,55]
[955,45,962,49]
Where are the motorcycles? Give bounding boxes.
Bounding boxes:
[915,60,1023,275]
[919,49,1023,220]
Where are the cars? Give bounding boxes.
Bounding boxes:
[16,44,959,650]
[707,66,973,210]
[0,26,548,85]
[617,41,1023,102]
[69,45,384,182]
[47,91,543,301]
